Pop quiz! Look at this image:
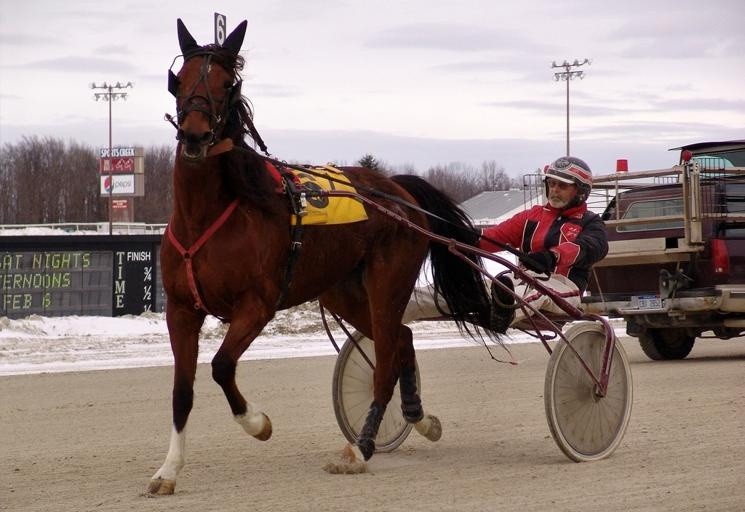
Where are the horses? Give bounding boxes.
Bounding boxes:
[144,17,513,498]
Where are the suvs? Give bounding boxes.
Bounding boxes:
[545,138,745,362]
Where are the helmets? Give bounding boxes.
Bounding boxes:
[542,156,592,189]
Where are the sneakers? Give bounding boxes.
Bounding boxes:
[492,277,514,332]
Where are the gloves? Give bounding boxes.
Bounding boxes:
[519,249,558,274]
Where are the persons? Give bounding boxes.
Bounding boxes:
[402,155,609,333]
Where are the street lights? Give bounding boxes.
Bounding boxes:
[90,81,134,235]
[550,56,591,156]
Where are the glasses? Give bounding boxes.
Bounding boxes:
[547,179,573,189]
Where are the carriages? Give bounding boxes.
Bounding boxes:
[135,16,635,502]
